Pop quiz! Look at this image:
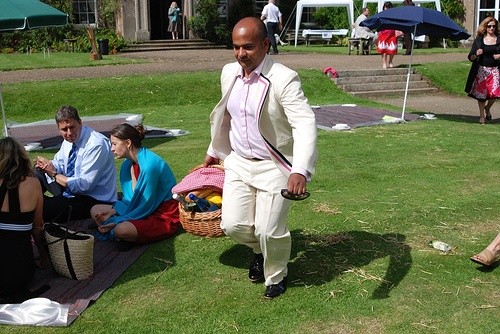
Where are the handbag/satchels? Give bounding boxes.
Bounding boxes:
[32,164,63,209]
[35,204,94,280]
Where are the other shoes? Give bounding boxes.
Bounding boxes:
[383,63,387,68]
[484,106,491,120]
[403,49,411,55]
[479,116,486,123]
[116,240,135,251]
[389,62,394,68]
[272,49,278,54]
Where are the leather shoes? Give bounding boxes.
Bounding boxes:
[248,249,264,281]
[264,274,288,298]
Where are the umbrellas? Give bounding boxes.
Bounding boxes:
[359,7,471,118]
[0,0,68,137]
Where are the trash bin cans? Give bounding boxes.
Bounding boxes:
[97,39,109,55]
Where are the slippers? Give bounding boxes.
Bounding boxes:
[470,248,500,266]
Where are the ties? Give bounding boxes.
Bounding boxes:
[65,142,77,196]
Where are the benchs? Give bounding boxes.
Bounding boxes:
[286,29,330,46]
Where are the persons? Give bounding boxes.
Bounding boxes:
[377,1,398,69]
[400,0,415,55]
[263,19,288,46]
[0,137,50,304]
[204,17,317,297]
[168,2,183,39]
[464,17,500,125]
[90,123,180,243]
[260,0,282,55]
[350,23,355,51]
[34,105,117,223]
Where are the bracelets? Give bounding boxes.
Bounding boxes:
[51,172,59,182]
[475,53,479,57]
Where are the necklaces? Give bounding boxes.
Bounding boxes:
[355,8,375,55]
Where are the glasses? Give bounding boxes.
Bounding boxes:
[487,25,496,28]
[281,188,310,201]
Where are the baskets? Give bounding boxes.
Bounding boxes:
[178,162,225,236]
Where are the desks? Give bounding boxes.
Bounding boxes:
[63,39,77,53]
[302,29,348,46]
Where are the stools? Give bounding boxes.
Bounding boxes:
[348,37,372,55]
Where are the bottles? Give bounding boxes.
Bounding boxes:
[172,194,202,214]
[189,193,219,212]
[432,240,452,252]
